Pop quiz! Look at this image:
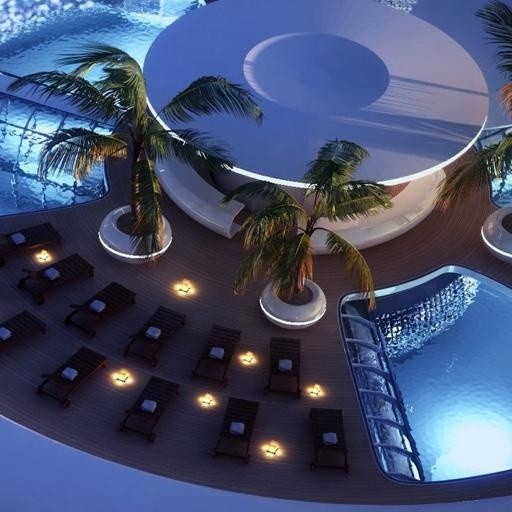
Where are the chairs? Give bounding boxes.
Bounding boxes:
[191,324,242,386]
[37,346,109,409]
[1,223,62,265]
[259,336,303,397]
[17,253,95,305]
[116,375,181,442]
[210,396,260,466]
[64,282,138,341]
[124,305,186,368]
[308,406,351,473]
[2,309,46,356]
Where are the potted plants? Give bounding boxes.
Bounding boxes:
[432,0,512,267]
[5,39,264,266]
[215,138,393,333]
